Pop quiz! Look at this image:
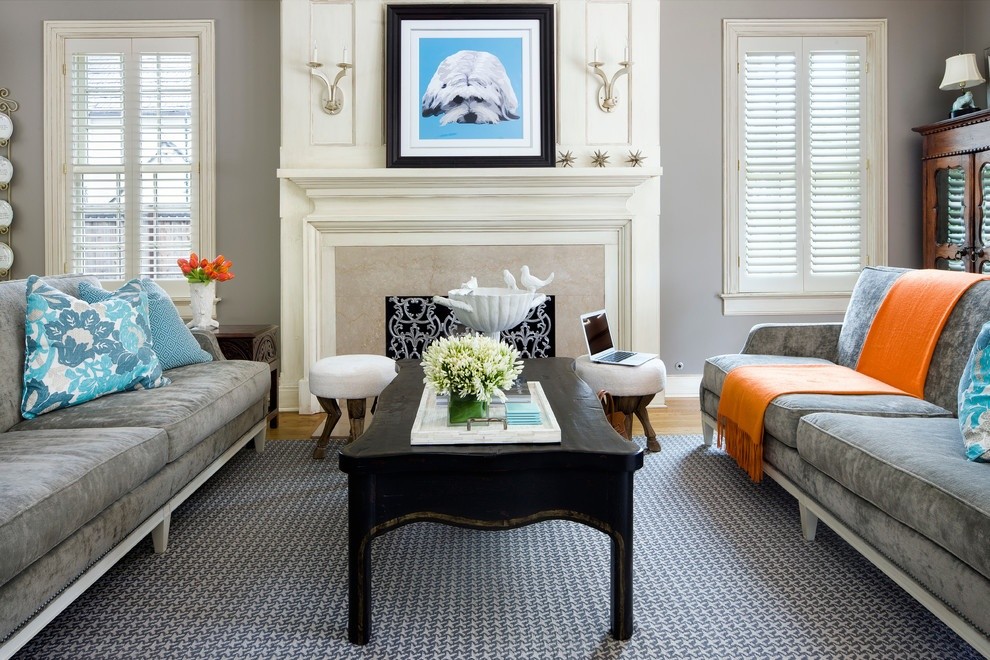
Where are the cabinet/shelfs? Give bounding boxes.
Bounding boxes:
[910,107,990,275]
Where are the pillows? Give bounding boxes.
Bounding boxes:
[78,277,213,370]
[957,321,990,465]
[21,274,172,418]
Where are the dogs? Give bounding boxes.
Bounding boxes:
[421,49,521,127]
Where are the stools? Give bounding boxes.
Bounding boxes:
[577,355,666,452]
[309,355,398,459]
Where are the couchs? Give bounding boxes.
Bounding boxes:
[0,272,271,660]
[699,263,990,660]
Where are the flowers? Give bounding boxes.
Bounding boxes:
[418,330,526,399]
[176,253,236,283]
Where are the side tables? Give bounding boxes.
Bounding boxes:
[211,324,281,429]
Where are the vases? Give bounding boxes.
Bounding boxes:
[447,387,490,427]
[432,264,554,343]
[185,280,216,332]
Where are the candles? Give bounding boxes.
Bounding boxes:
[624,46,628,62]
[313,44,317,61]
[343,47,347,63]
[594,43,598,62]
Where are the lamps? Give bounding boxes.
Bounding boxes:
[938,52,986,118]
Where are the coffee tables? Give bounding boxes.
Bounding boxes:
[337,354,645,644]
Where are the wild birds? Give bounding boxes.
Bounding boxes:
[459,277,478,290]
[503,265,554,291]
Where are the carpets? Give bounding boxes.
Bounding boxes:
[11,431,986,660]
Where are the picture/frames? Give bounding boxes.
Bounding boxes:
[384,2,556,169]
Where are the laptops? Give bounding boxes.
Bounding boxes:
[580,309,658,366]
